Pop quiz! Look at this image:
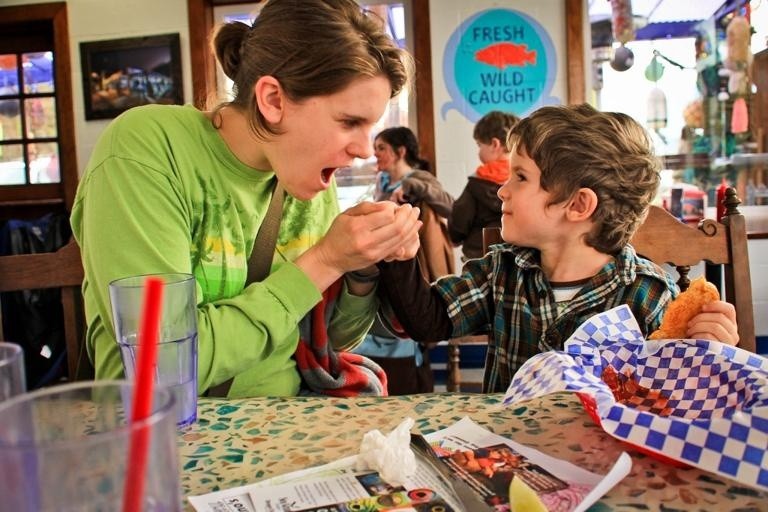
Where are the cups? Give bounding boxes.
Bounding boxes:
[106,272,200,432]
[0,374,181,512]
[0,342,27,403]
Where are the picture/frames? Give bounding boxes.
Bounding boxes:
[78,32,185,123]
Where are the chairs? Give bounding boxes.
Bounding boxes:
[0,234,84,398]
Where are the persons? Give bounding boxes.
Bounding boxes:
[447,111,520,261]
[376,100,743,395]
[70,2,424,403]
[372,125,456,218]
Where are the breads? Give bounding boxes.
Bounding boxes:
[647,276,720,340]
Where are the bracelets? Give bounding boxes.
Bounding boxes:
[348,271,381,282]
